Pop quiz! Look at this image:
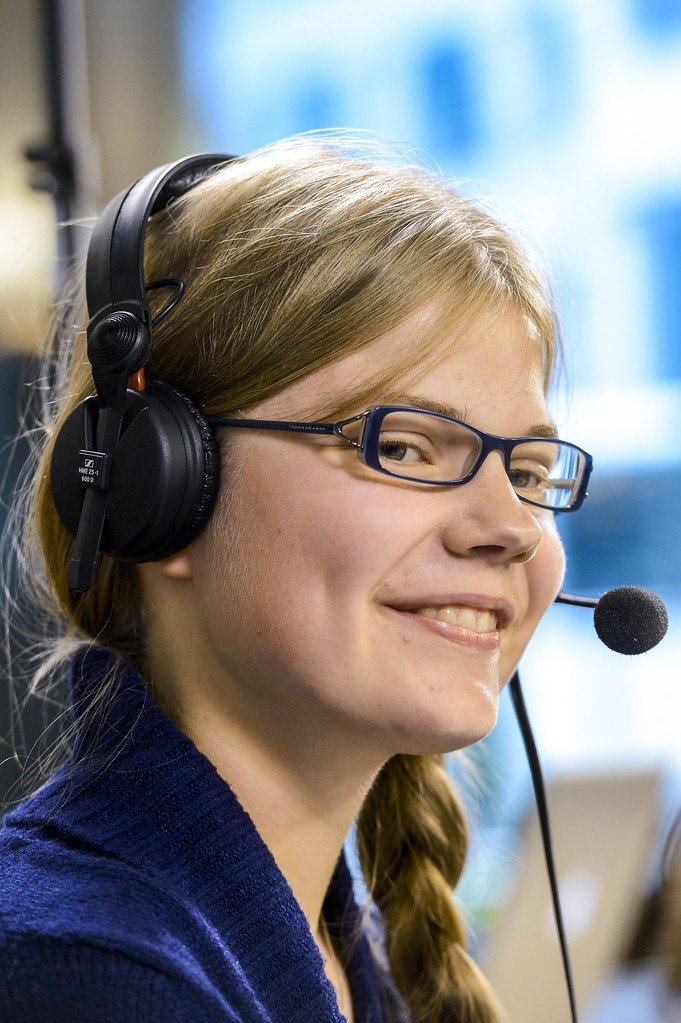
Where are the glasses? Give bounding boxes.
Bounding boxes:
[208,406,593,514]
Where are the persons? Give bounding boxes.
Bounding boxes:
[0,129,567,1023]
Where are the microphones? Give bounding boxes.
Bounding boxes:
[555,585,667,657]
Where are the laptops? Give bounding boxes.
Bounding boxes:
[480,766,666,1023]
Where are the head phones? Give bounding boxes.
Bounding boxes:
[49,153,240,606]
